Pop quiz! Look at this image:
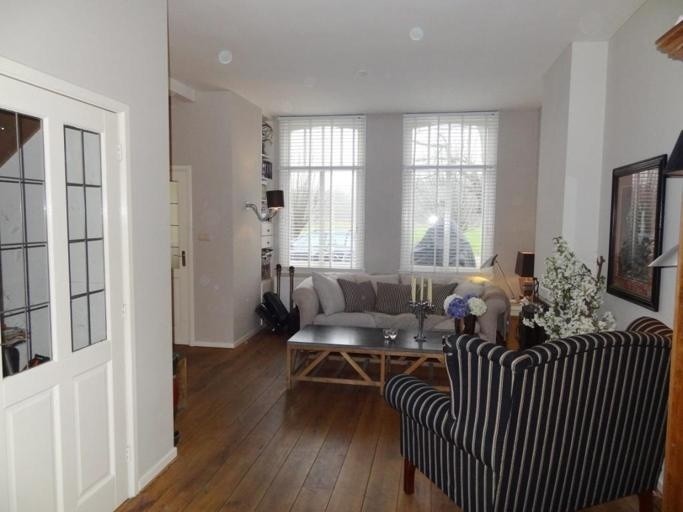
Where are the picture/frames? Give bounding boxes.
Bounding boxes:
[606,153,668,311]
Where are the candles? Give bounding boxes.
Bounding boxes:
[410,276,433,307]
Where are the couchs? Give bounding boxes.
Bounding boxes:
[290,274,508,344]
[382,316,673,511]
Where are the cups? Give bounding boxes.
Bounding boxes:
[382,328,399,340]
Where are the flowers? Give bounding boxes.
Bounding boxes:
[523,233,617,343]
[443,292,488,319]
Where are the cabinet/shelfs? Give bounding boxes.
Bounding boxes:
[653,19,683,512]
[260,113,275,326]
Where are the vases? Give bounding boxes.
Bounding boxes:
[454,316,476,335]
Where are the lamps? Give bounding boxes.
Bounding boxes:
[514,250,534,277]
[491,253,517,299]
[244,189,284,222]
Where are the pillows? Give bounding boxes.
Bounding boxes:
[336,278,377,313]
[452,277,491,299]
[374,281,418,313]
[416,278,458,316]
[311,273,346,316]
